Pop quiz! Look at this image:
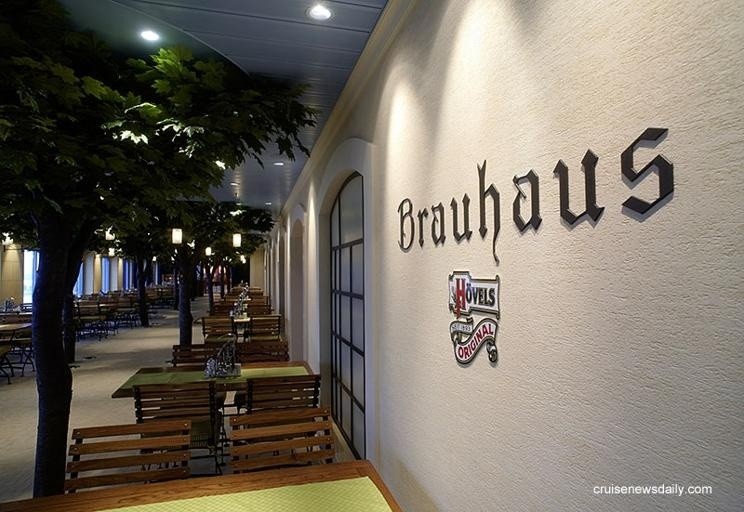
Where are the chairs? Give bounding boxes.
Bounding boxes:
[0,287,335,493]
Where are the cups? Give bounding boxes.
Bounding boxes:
[243,312,247,319]
[234,364,241,376]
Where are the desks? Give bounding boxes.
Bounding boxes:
[0,459,402,512]
